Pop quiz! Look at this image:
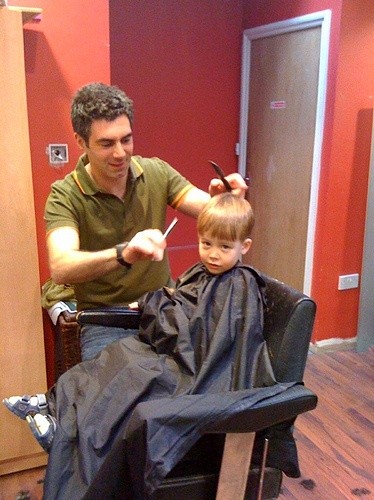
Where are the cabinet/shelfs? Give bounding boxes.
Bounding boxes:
[0,6,68,477]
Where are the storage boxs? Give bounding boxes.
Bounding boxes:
[53,299,83,383]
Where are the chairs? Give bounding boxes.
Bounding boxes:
[74,274,317,500]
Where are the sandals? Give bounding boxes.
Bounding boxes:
[25,414,56,454]
[3,394,48,420]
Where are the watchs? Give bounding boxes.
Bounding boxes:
[115,242,133,270]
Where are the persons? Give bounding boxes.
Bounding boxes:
[43,82,249,362]
[1,192,256,456]
